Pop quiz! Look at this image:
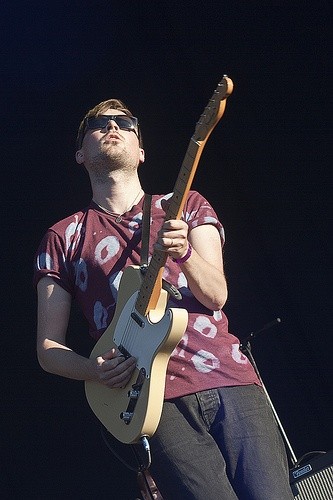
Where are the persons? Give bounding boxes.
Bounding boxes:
[33,100,296,500]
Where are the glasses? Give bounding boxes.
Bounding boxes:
[79,114,142,149]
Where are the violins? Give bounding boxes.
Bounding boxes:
[84,74,235,444]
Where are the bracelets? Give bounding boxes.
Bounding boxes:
[171,243,193,264]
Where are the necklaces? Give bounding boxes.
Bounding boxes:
[93,188,142,223]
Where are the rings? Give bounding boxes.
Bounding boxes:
[170,237,173,247]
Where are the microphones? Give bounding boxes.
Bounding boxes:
[238,332,256,351]
